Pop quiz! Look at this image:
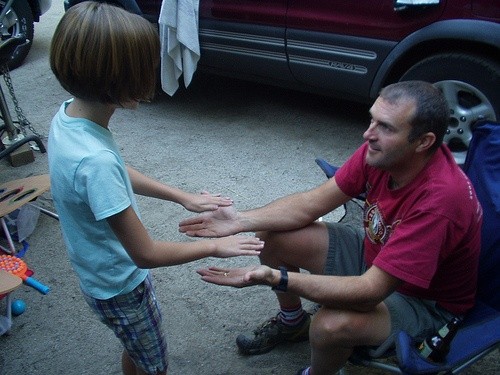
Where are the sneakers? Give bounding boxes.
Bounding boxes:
[236,312,314,354]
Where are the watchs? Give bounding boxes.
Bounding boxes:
[271,266,288,293]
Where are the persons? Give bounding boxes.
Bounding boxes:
[179,79,483,375]
[48,1,264,375]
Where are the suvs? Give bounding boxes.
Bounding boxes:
[62,0,499,170]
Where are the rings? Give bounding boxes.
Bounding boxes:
[224,272,227,276]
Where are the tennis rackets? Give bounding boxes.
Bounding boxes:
[0,255,51,294]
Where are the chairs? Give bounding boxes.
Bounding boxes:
[314,118,500,375]
[0,134,59,294]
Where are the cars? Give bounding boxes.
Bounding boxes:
[0,0,52,77]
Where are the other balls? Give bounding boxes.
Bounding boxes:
[11,299,26,315]
